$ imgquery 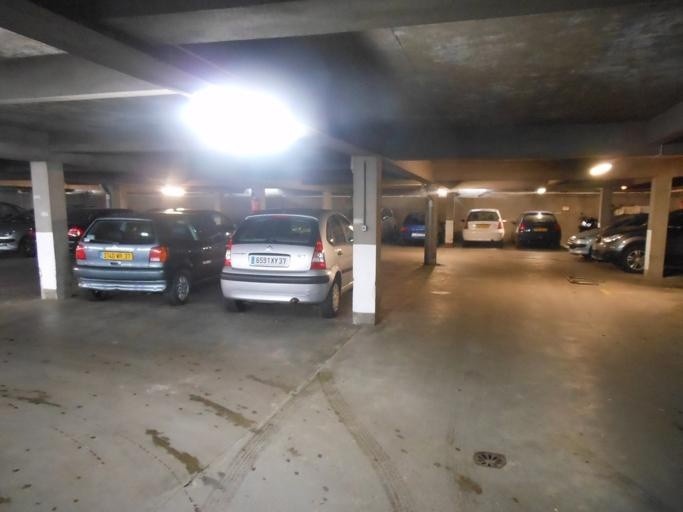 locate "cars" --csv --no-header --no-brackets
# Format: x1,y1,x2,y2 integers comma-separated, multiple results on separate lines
380,210,446,247
219,206,353,319
0,202,236,308
460,208,507,249
509,209,562,249
567,208,683,274
